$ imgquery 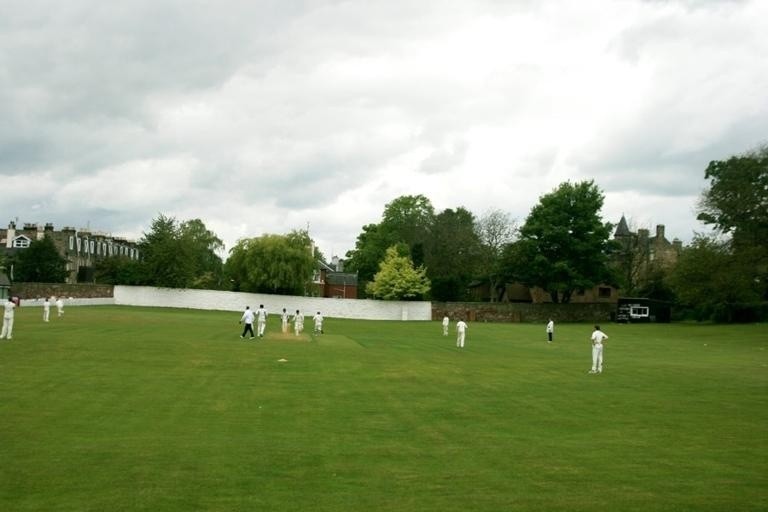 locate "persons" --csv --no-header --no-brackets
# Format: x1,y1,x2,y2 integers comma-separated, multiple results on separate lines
255,304,268,337
0,296,16,340
588,325,609,374
280,308,289,332
238,306,256,339
546,317,554,343
442,313,450,336
313,312,324,337
455,318,468,348
42,298,51,322
292,309,304,336
55,298,65,317
